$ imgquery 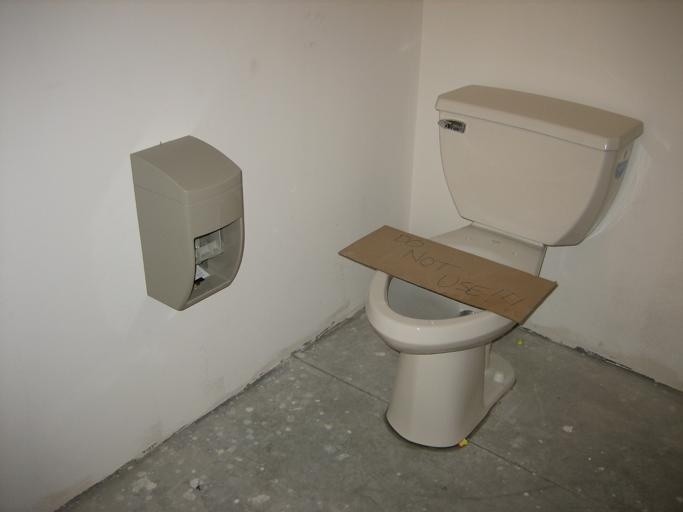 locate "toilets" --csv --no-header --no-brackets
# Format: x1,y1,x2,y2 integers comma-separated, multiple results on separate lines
362,81,646,451
